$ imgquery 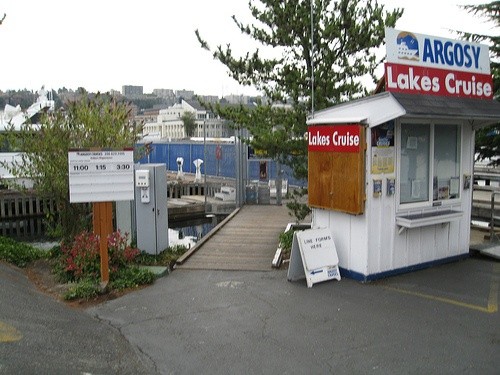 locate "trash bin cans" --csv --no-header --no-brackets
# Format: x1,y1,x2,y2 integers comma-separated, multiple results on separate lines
246,185,257,204
257,185,271,205
